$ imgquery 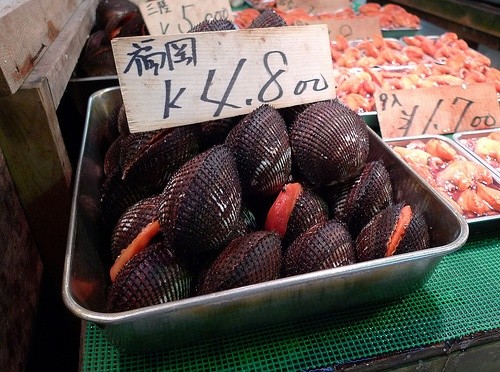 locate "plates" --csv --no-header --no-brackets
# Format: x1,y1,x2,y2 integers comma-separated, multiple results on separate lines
328,36,500,223
